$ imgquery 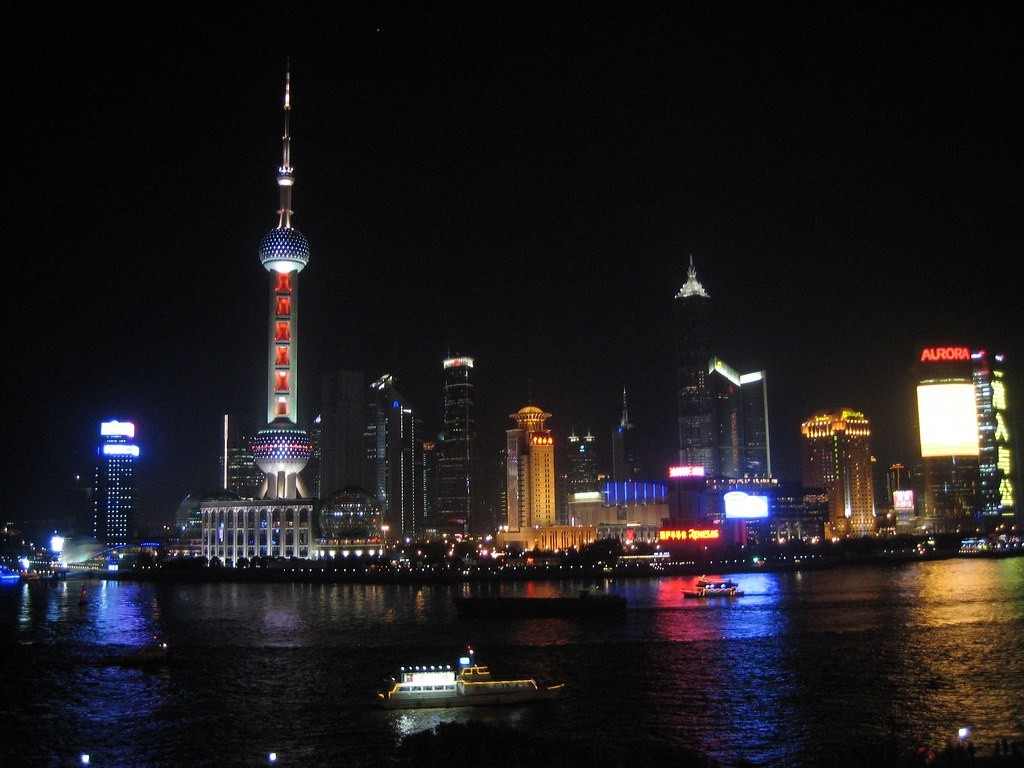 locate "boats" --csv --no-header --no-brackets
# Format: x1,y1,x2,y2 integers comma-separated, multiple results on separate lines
682,578,744,598
376,646,569,705
594,554,686,577
958,532,1024,558
